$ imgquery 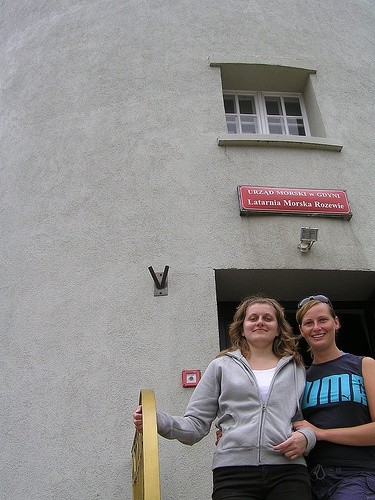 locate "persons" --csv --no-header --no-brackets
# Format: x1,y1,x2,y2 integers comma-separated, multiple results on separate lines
132,295,316,500
216,295,375,500
